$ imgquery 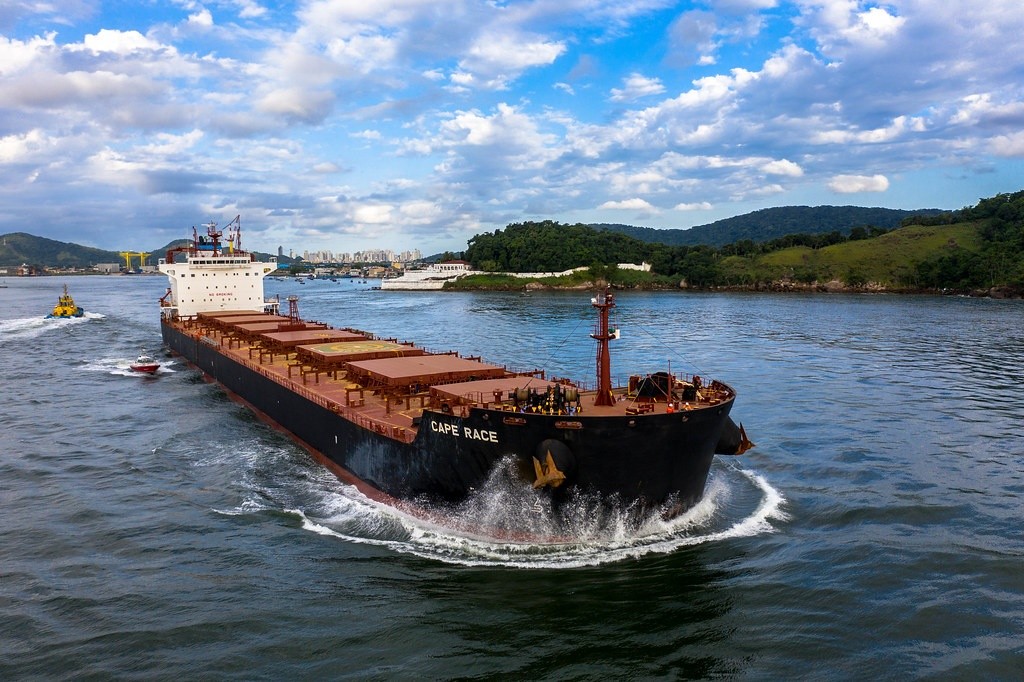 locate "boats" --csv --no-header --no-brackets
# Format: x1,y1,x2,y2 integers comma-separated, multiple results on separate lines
43,283,84,320
0,280,8,289
262,273,367,285
129,348,160,375
157,213,759,546
